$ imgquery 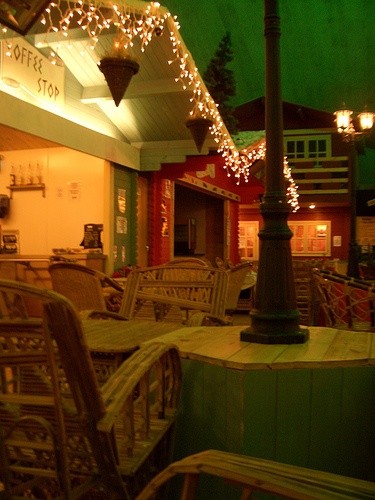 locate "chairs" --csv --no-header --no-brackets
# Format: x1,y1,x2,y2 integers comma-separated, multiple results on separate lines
0,253,375,500
